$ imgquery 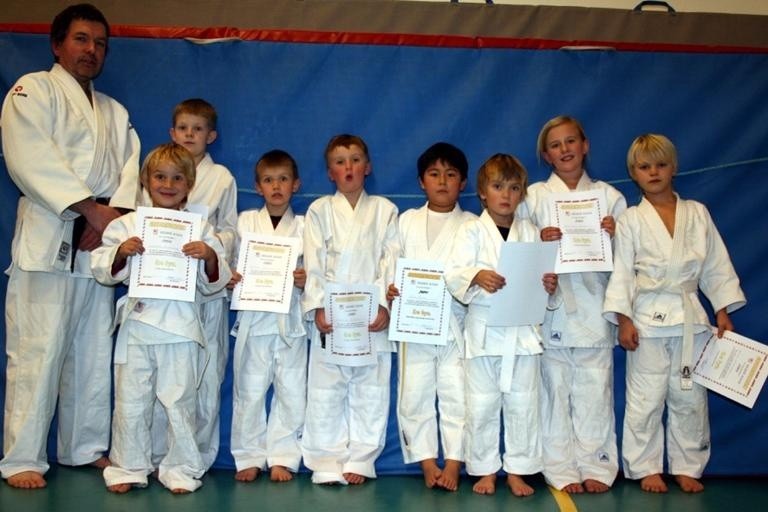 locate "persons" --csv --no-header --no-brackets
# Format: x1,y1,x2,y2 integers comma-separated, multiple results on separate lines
89,141,235,497
516,113,628,495
141,96,238,267
601,133,749,495
385,139,482,490
441,151,560,497
301,132,400,488
1,3,141,491
226,148,309,484
